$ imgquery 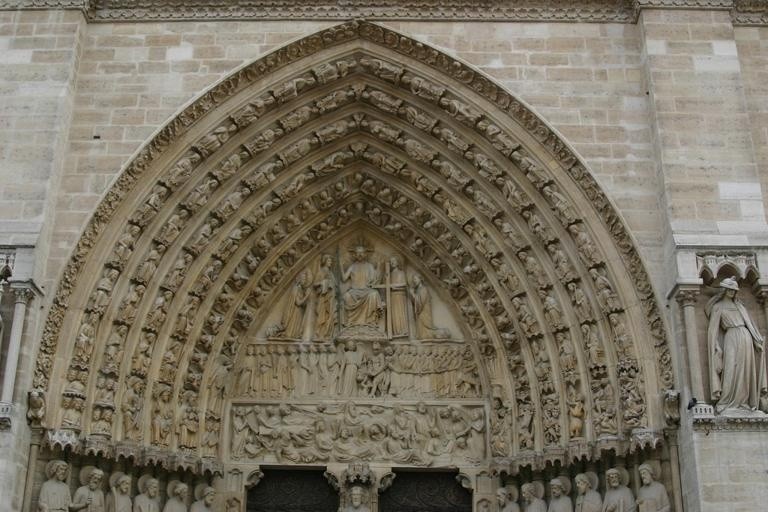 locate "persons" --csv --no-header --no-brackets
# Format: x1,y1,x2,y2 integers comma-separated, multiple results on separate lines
38,52,673,512
705,274,766,416
